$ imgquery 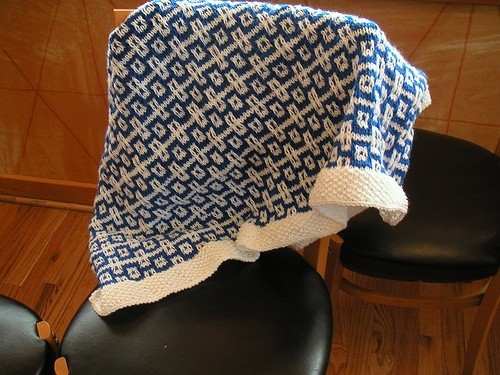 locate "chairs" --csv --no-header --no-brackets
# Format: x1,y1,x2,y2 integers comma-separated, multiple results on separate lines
323,129,498,374
0,292,62,374
54,0,431,374
